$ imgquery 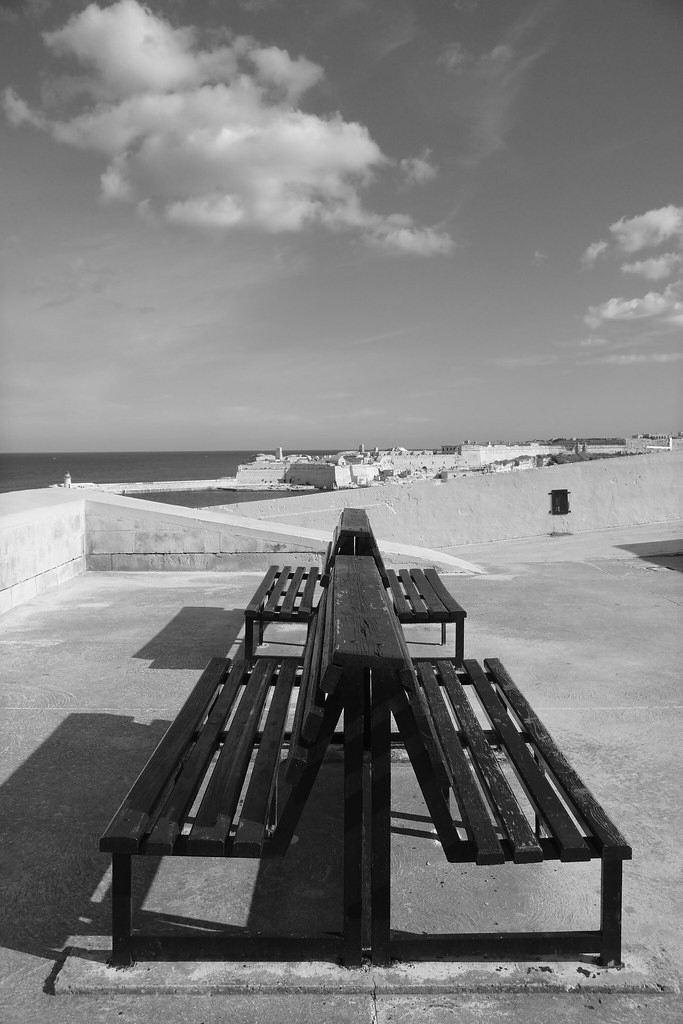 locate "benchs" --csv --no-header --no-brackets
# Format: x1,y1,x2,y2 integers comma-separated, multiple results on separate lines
95,509,633,968
